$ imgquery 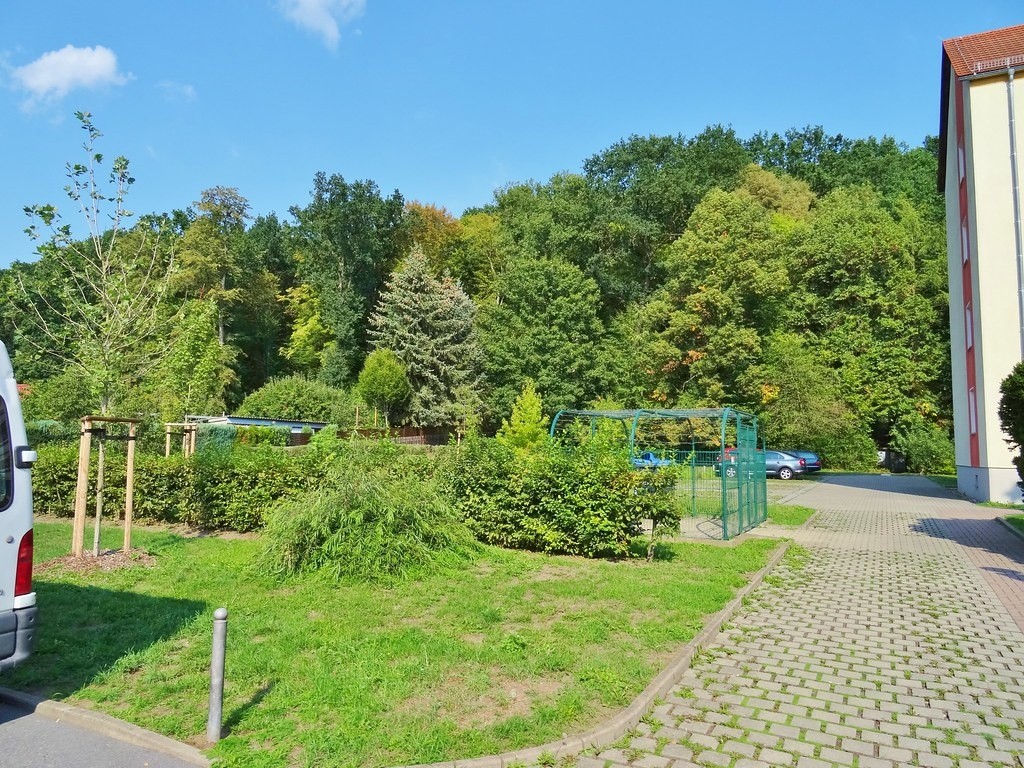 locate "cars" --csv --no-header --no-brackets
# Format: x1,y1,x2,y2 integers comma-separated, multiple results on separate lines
0,342,37,687
628,452,679,469
716,446,761,462
713,450,807,479
784,451,822,474
